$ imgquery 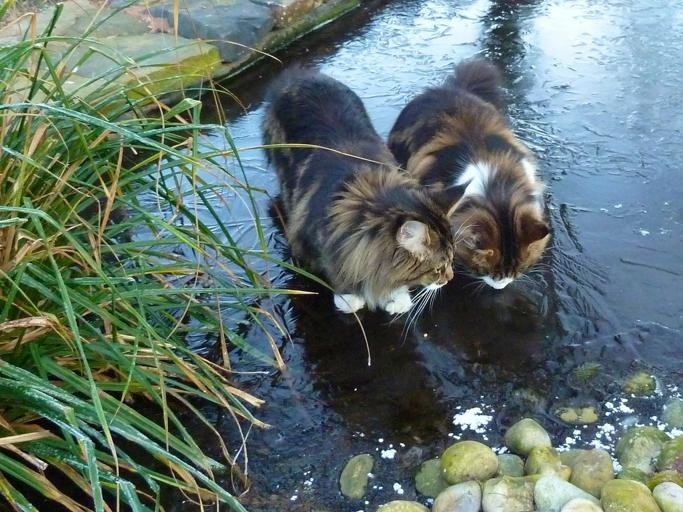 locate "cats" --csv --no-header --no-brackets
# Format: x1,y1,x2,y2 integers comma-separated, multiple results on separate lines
388,58,563,294
259,62,480,348
